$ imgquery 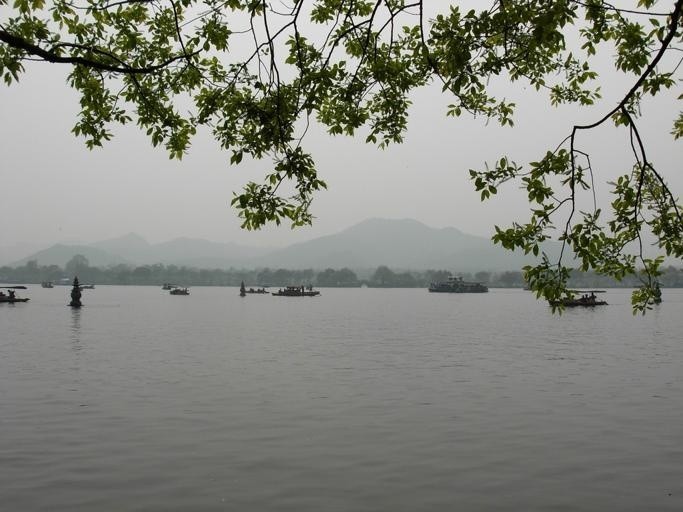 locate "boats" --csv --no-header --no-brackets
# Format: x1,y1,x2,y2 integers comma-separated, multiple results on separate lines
548,291,609,306
161,282,191,296
425,275,489,293
271,285,320,296
40,280,55,289
79,281,96,289
0,284,32,304
522,284,529,290
244,288,270,293
